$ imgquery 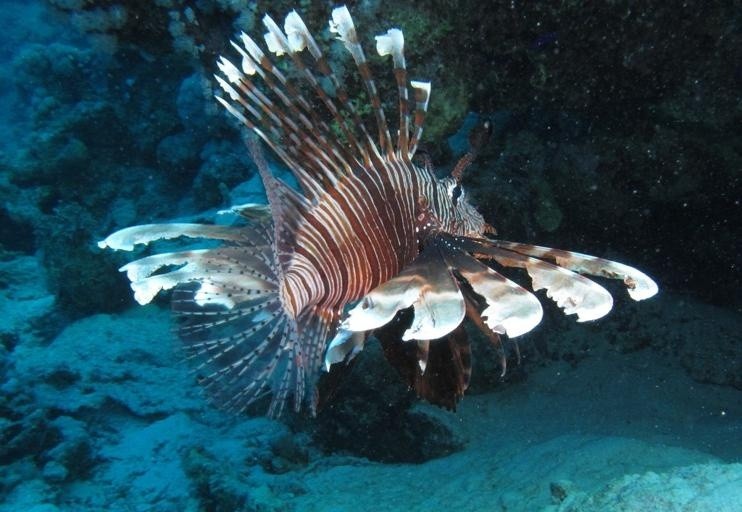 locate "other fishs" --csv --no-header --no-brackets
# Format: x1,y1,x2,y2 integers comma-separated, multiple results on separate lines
95,4,661,420
